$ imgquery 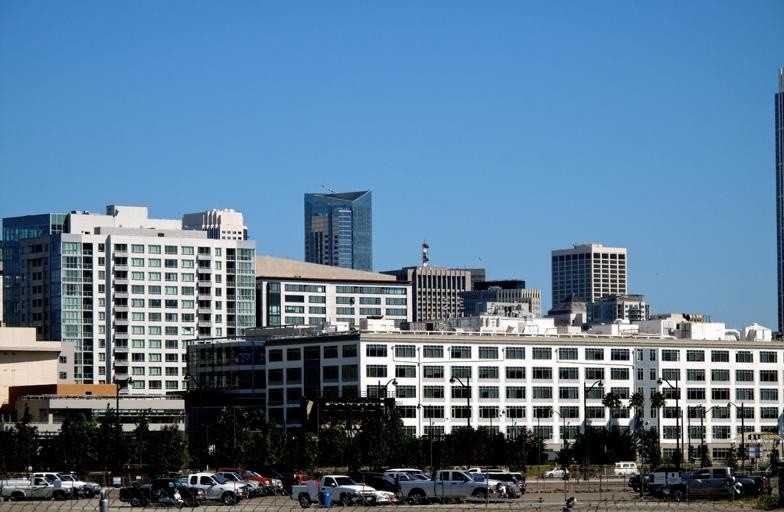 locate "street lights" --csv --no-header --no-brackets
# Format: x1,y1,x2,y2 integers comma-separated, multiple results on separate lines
688,397,750,462
181,373,212,468
375,375,401,463
581,378,604,432
487,399,572,465
656,370,681,464
415,400,435,468
447,371,474,434
110,376,135,482
